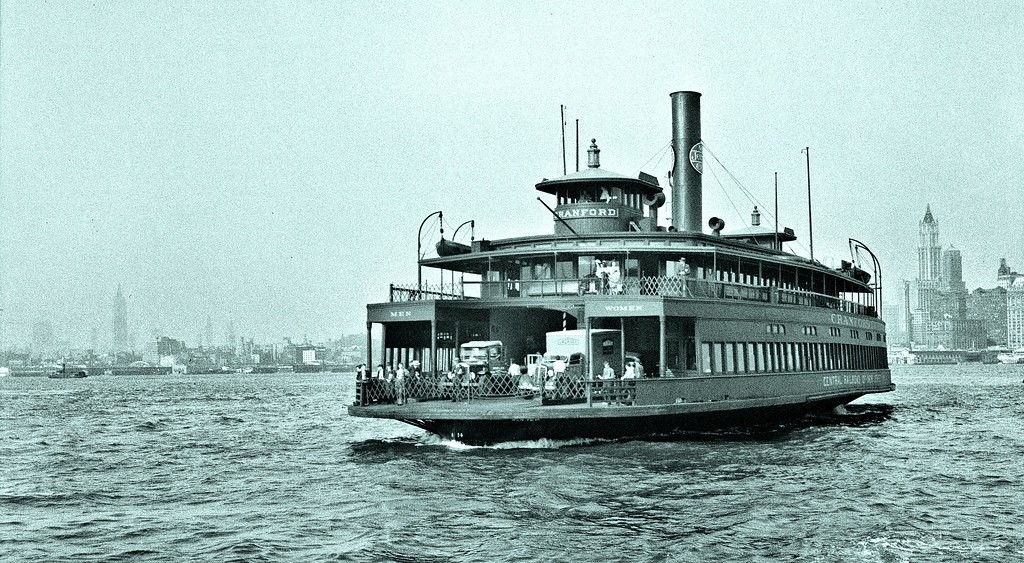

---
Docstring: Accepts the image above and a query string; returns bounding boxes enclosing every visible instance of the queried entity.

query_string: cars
[442,340,509,394]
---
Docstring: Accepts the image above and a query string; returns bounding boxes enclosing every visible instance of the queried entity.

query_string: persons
[449,358,463,402]
[594,259,622,295]
[356,360,421,406]
[553,356,566,400]
[598,361,615,405]
[620,358,644,406]
[677,258,690,296]
[508,360,521,393]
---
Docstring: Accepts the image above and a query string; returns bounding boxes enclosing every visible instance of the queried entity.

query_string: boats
[49,362,87,379]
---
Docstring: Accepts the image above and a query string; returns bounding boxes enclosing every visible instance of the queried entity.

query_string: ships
[348,89,898,447]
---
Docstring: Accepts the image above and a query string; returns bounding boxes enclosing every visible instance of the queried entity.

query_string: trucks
[517,327,623,399]
